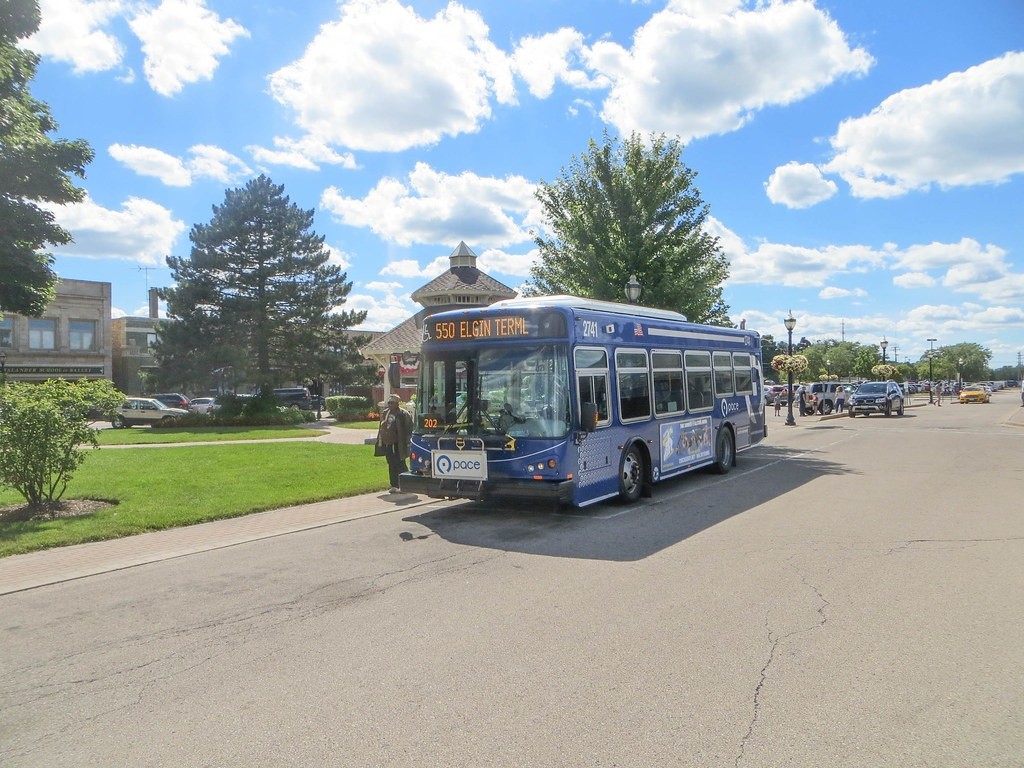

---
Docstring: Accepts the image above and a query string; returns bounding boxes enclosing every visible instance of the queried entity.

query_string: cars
[960,384,989,404]
[858,380,1019,396]
[310,394,325,412]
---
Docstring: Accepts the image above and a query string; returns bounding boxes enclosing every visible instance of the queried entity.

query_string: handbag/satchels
[374,433,386,455]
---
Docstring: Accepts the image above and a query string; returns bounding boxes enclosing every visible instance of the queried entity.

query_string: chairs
[661,390,712,411]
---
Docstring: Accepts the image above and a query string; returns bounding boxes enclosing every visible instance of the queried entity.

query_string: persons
[799,387,807,417]
[1020,380,1024,407]
[775,393,780,416]
[374,395,414,494]
[810,392,818,415]
[934,381,941,406]
[834,386,845,413]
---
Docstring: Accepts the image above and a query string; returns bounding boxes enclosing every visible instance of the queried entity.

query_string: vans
[253,387,314,411]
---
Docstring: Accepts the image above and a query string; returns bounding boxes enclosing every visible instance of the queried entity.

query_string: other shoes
[389,487,403,493]
[934,403,935,405]
[1020,405,1024,407]
[938,405,941,406]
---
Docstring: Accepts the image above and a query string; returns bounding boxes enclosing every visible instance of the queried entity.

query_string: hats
[813,392,817,395]
[387,394,401,402]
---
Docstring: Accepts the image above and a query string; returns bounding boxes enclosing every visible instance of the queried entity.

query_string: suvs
[764,379,808,406]
[149,392,256,420]
[111,397,190,429]
[792,382,861,414]
[846,379,905,418]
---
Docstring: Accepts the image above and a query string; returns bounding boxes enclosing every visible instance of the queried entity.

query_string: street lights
[784,308,797,425]
[827,360,831,382]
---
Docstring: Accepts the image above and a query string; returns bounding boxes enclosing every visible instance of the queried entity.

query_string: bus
[388,294,767,510]
